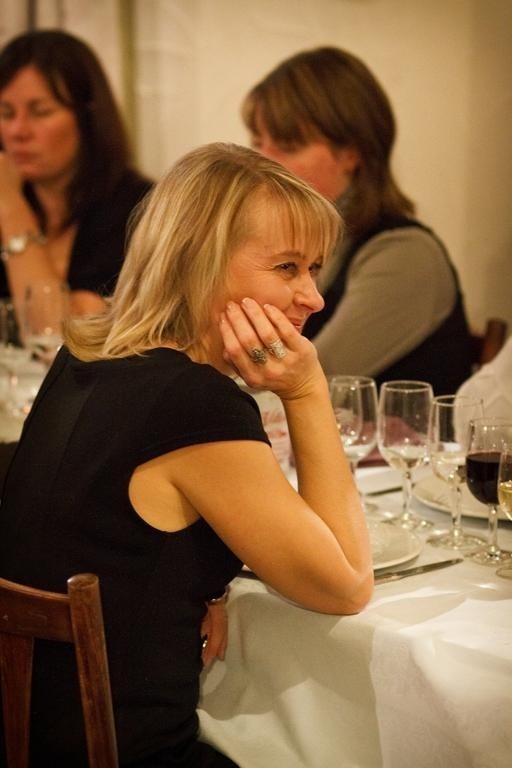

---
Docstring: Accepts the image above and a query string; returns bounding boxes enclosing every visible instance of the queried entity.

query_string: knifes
[374,558,463,585]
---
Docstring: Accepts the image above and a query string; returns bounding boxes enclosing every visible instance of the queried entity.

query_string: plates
[367,519,424,570]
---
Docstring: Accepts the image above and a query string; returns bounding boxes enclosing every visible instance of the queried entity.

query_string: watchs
[0,232,35,262]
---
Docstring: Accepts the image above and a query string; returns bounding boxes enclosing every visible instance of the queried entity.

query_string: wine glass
[330,376,378,515]
[0,281,70,419]
[376,381,512,580]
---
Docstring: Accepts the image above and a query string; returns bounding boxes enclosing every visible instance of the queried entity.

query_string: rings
[250,347,268,364]
[269,337,287,358]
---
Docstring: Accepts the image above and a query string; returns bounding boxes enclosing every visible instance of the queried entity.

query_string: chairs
[0,567,124,766]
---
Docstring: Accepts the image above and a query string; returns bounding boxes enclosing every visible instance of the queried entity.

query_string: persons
[0,29,158,365]
[239,46,470,447]
[0,141,376,768]
